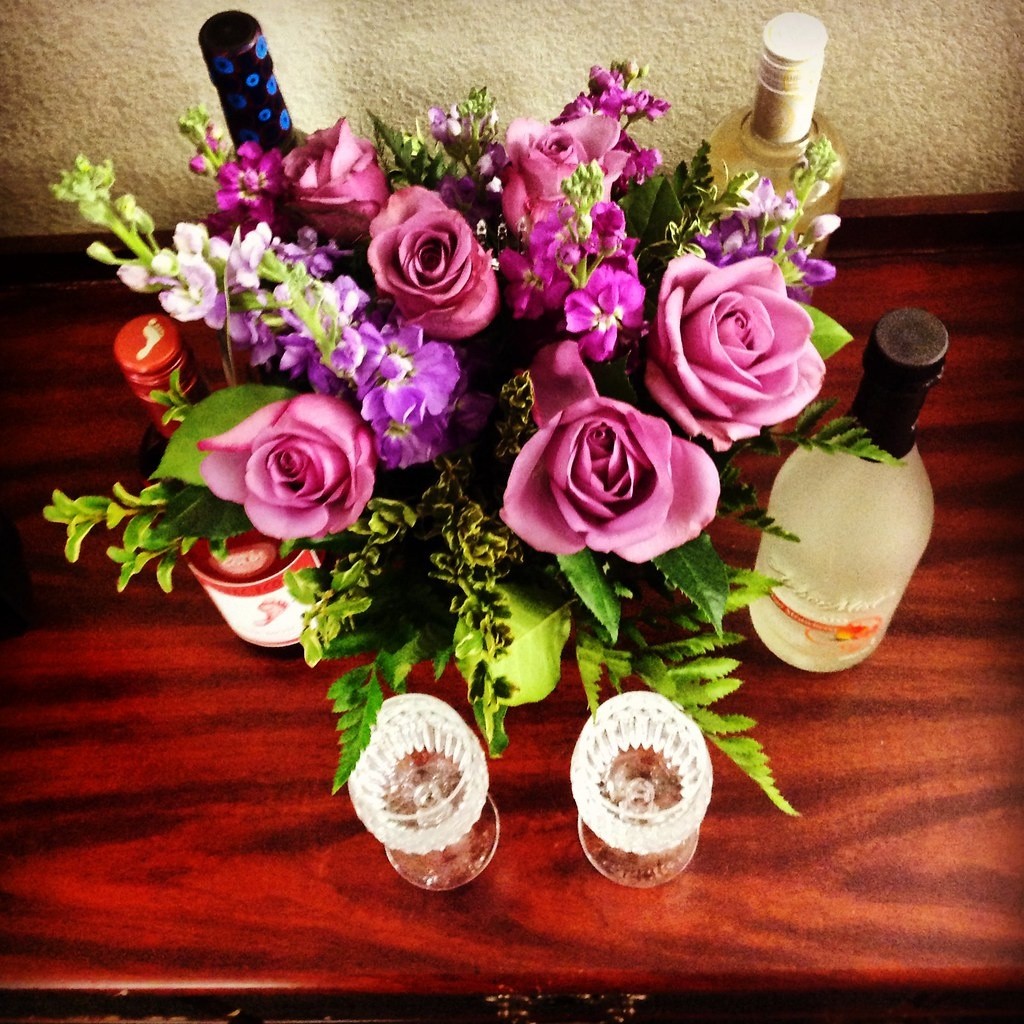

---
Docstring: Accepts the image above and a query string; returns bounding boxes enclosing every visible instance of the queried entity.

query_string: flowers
[42,57,902,819]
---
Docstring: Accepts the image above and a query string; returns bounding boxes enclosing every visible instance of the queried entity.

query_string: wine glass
[345,695,500,893]
[569,689,713,890]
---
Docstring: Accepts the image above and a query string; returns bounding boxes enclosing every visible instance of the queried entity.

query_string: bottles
[704,11,844,264]
[198,10,309,161]
[748,307,951,675]
[110,313,333,657]
